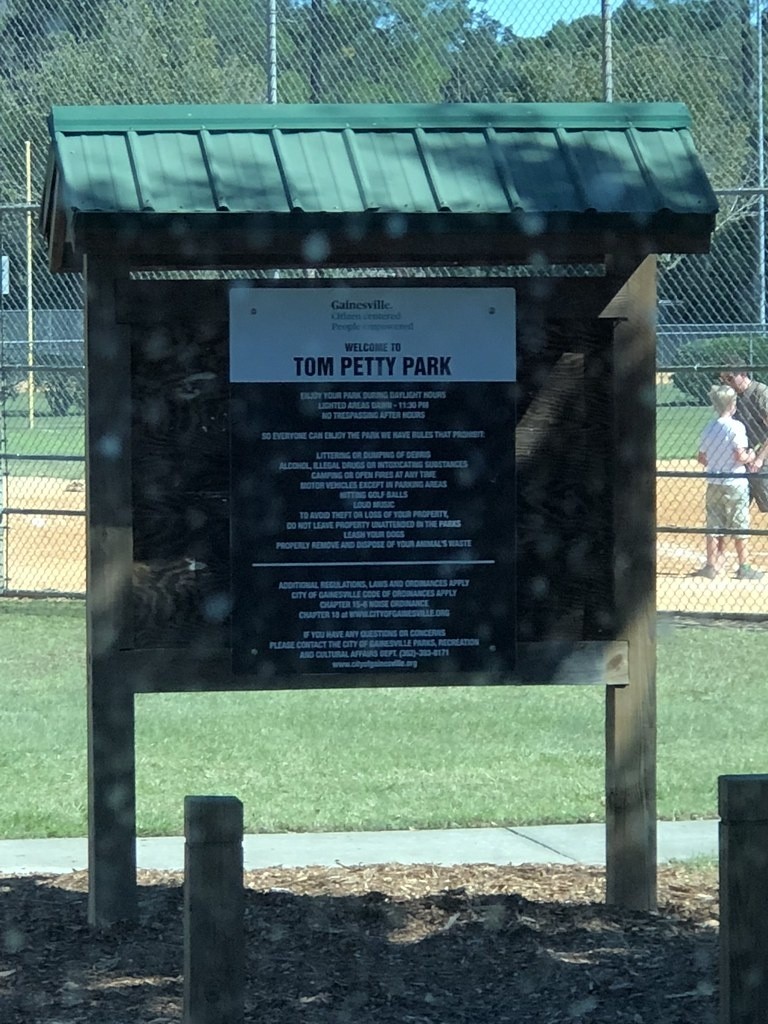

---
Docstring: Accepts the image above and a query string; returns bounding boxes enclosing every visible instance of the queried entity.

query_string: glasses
[717,376,738,385]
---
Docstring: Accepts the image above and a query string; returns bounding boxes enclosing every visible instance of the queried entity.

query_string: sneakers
[702,564,717,580]
[736,565,765,580]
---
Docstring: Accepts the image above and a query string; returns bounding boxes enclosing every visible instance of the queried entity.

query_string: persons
[716,357,768,580]
[696,384,765,580]
